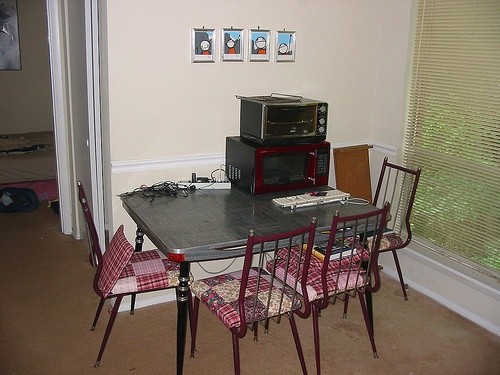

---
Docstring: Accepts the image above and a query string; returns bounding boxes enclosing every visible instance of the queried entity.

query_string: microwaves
[225,135,330,194]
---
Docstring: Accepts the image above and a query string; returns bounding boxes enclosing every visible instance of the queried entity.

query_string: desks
[120,185,392,375]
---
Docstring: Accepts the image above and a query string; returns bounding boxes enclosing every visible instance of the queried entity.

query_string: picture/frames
[273,30,297,62]
[221,27,245,62]
[248,28,272,62]
[191,27,216,62]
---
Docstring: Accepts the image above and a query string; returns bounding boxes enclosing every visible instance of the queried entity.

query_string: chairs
[190,217,317,375]
[327,157,421,302]
[266,201,391,375]
[77,181,197,369]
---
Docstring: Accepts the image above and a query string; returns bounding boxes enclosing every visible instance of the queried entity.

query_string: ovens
[240,94,328,145]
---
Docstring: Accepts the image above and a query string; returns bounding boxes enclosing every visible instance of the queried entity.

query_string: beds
[0,131,58,203]
